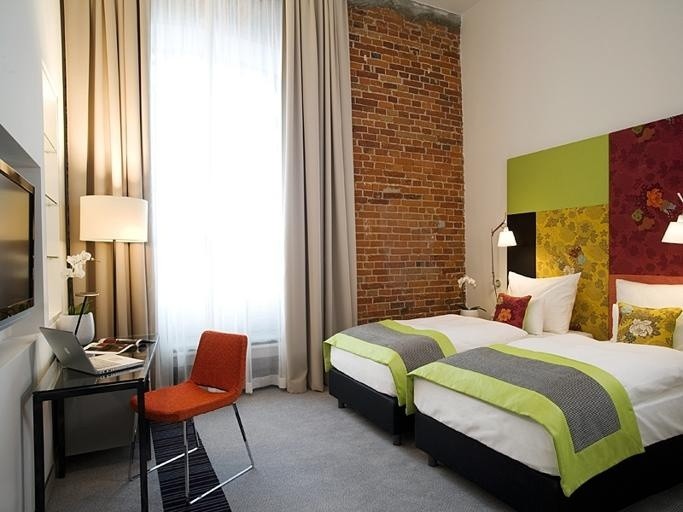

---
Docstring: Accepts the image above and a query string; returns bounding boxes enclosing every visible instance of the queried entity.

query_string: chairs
[128,331,254,506]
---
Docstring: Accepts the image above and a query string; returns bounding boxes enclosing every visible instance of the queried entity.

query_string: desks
[31,334,160,511]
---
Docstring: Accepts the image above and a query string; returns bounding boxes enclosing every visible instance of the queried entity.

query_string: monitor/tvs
[0,158,35,332]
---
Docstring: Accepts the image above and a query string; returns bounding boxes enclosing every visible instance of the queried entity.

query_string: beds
[406,273,683,512]
[321,313,593,446]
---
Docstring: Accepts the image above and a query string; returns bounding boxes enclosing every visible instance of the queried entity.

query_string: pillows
[614,279,682,349]
[616,303,682,349]
[610,302,618,343]
[521,295,548,336]
[507,270,581,336]
[492,293,532,329]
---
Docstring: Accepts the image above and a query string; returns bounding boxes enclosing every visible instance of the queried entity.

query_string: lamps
[78,195,147,340]
[660,192,682,245]
[488,214,517,295]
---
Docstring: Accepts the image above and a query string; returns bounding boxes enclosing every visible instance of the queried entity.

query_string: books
[82,339,156,354]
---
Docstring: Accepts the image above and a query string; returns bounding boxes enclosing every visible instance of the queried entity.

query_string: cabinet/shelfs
[43,132,58,260]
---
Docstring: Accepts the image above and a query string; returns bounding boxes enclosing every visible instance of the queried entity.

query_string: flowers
[63,250,91,315]
[454,275,488,313]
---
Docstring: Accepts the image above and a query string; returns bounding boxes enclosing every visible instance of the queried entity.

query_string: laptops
[40,326,145,375]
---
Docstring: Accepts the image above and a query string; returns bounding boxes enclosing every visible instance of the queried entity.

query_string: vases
[56,313,95,345]
[458,309,478,318]
